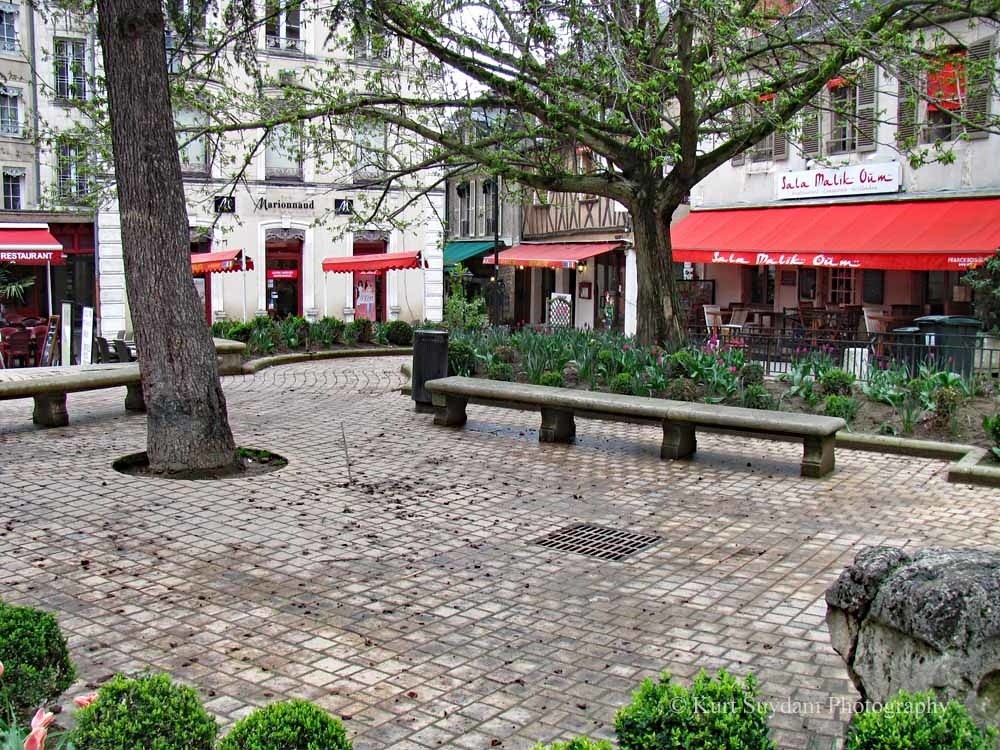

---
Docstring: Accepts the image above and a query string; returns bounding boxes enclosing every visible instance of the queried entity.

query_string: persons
[357,279,374,314]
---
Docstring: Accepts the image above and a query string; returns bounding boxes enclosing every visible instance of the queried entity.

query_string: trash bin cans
[914,316,984,378]
[892,326,921,381]
[412,329,449,408]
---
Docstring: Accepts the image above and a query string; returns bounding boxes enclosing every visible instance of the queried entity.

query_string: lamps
[577,261,584,275]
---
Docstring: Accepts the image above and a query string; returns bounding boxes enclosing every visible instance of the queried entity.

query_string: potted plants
[959,247,1000,369]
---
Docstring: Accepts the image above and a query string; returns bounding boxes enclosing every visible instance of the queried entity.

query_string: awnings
[0,224,66,265]
[670,195,1000,272]
[443,242,503,266]
[191,248,254,326]
[482,242,623,269]
[322,249,425,325]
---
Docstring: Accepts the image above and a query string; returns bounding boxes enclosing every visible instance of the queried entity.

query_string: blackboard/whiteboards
[39,315,60,366]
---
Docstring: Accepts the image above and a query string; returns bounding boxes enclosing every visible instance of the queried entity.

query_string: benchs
[424,375,845,479]
[0,362,147,426]
[212,337,245,376]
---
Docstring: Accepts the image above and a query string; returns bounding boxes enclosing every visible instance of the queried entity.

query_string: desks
[98,340,137,357]
[868,314,910,354]
[706,308,785,332]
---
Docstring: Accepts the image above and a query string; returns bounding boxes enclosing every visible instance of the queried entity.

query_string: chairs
[94,329,134,362]
[0,311,48,368]
[703,300,897,359]
